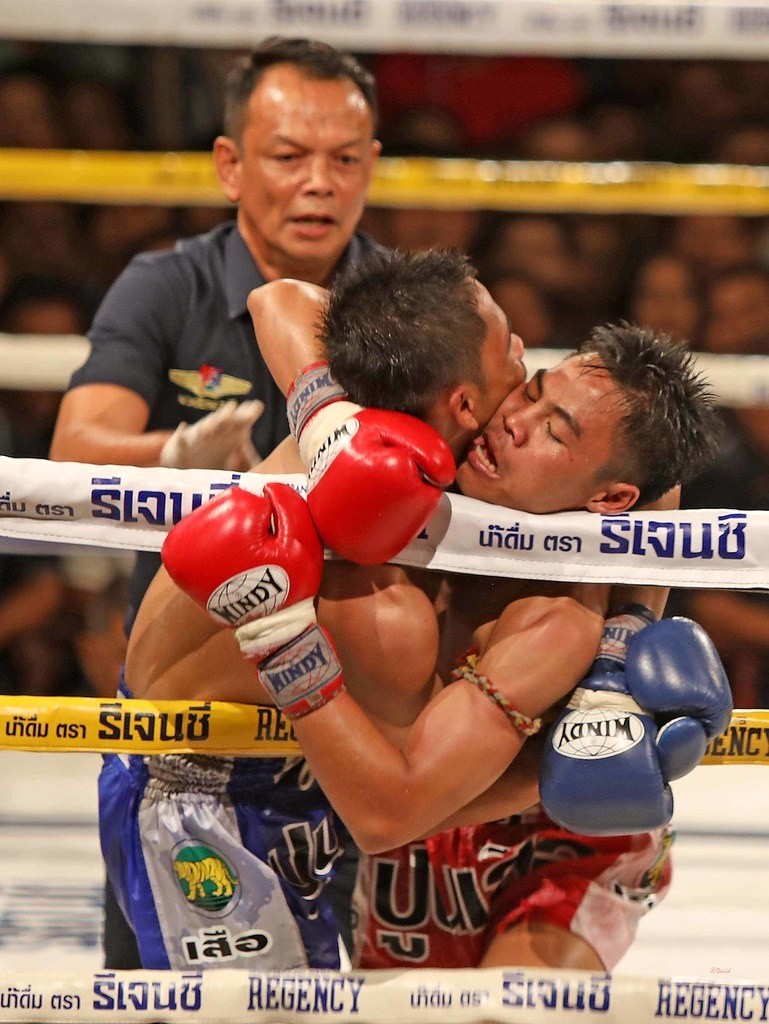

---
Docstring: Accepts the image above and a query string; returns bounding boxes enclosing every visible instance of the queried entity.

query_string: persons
[97,247,737,969]
[0,34,769,709]
[49,34,392,968]
[160,279,723,968]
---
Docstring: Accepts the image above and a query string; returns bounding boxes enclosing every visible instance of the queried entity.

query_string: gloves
[162,480,327,655]
[537,612,675,837]
[298,397,458,565]
[622,615,731,785]
